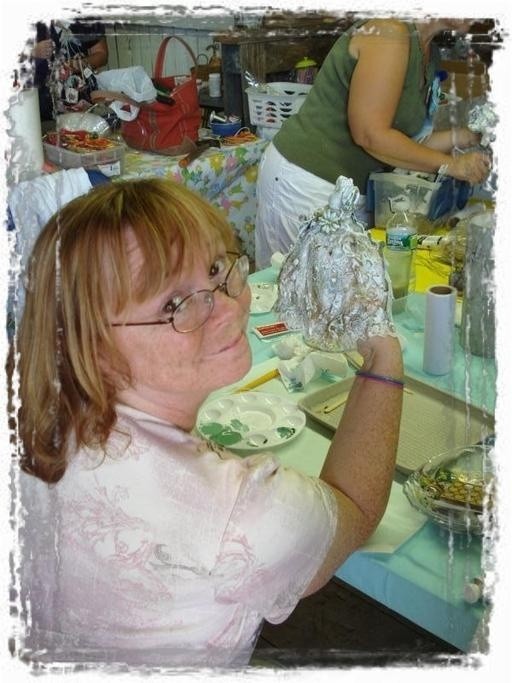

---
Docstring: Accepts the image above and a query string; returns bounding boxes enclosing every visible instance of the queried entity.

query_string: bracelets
[354,371,406,387]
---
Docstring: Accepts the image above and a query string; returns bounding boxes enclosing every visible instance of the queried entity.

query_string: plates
[403,443,494,536]
[195,391,307,453]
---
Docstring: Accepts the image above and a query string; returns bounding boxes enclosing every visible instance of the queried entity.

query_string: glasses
[105,252,250,334]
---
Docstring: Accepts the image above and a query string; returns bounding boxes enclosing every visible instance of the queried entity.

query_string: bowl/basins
[211,122,242,136]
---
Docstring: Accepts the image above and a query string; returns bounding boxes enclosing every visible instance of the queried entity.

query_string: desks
[110,118,266,263]
[193,259,484,652]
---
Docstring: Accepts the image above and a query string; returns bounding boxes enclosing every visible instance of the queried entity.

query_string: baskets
[245,82,313,128]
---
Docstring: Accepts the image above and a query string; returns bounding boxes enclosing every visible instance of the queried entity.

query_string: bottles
[381,199,415,315]
[208,74,222,98]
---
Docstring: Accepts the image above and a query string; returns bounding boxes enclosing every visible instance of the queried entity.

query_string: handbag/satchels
[90,36,202,156]
[94,65,157,121]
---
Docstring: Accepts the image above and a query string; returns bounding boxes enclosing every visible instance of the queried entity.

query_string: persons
[253,18,491,269]
[32,20,108,119]
[12,179,405,665]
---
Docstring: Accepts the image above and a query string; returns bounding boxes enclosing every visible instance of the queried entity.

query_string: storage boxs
[368,170,441,229]
